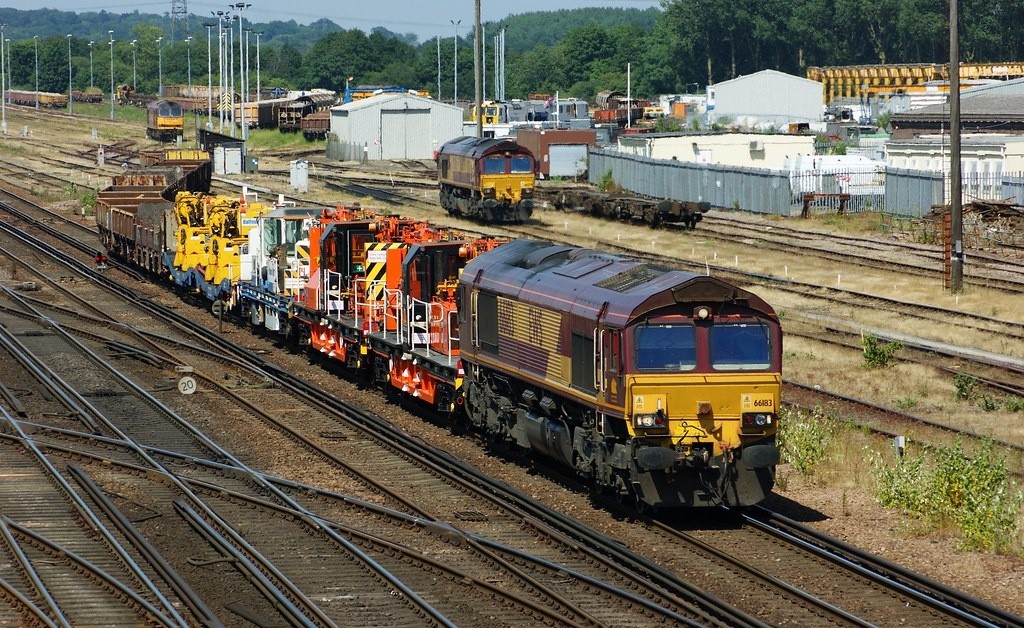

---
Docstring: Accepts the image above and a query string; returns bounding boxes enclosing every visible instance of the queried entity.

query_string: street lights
[480,22,489,102]
[34,36,39,109]
[253,31,265,101]
[185,37,193,99]
[130,40,137,91]
[0,23,8,130]
[5,39,11,104]
[88,41,94,88]
[109,31,116,119]
[67,34,72,113]
[229,2,252,140]
[202,23,217,131]
[451,20,461,108]
[211,11,230,134]
[156,37,163,97]
[243,27,254,102]
[492,25,509,101]
[220,15,238,138]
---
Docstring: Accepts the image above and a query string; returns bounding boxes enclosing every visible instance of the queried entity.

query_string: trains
[74,86,104,103]
[235,89,340,140]
[93,148,784,511]
[5,89,67,108]
[436,135,536,224]
[472,96,666,139]
[116,85,217,114]
[146,100,184,142]
